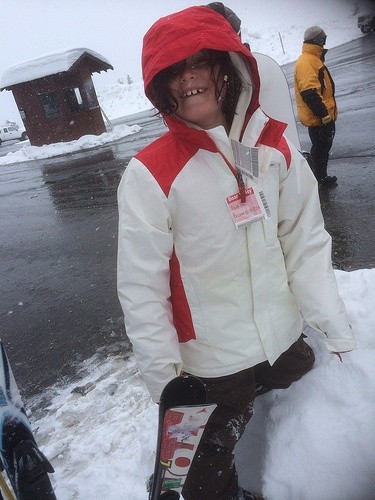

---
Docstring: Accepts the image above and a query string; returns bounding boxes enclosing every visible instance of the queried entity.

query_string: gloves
[320,114,335,139]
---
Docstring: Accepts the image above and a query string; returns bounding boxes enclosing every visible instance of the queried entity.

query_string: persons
[295,25,338,189]
[116,2,357,500]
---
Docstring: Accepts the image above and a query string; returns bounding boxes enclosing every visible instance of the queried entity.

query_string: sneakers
[319,174,338,184]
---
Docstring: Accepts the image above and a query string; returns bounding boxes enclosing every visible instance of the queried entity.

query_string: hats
[303,25,327,44]
